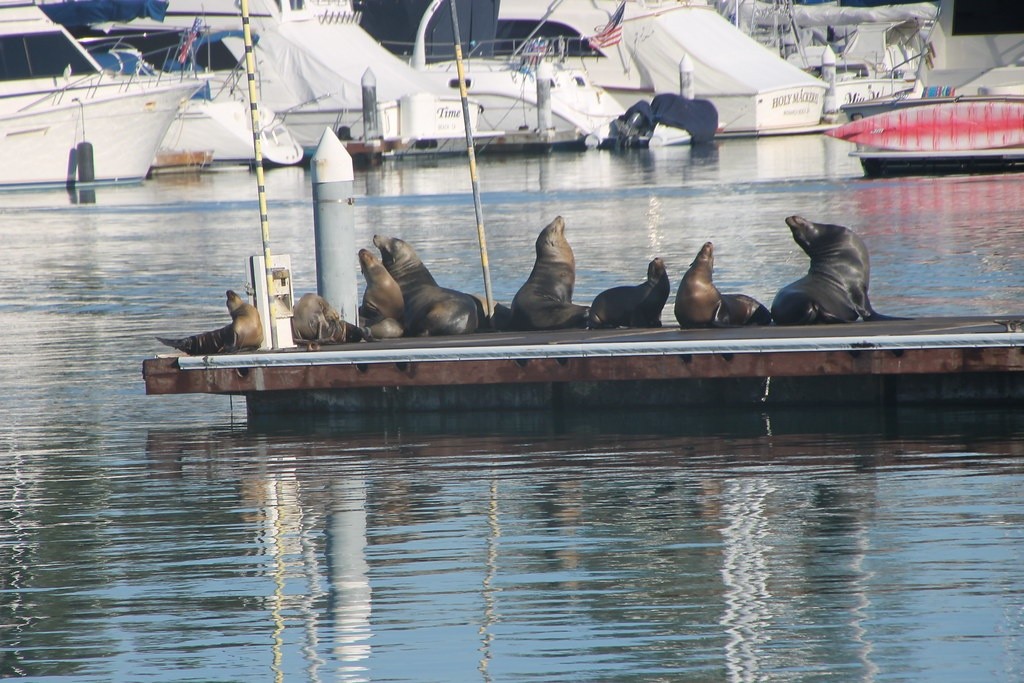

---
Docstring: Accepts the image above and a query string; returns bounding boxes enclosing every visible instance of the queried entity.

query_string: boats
[821,57,1024,178]
[0,2,944,193]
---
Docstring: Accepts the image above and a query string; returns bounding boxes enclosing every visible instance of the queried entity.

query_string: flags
[178,18,202,64]
[589,1,626,48]
